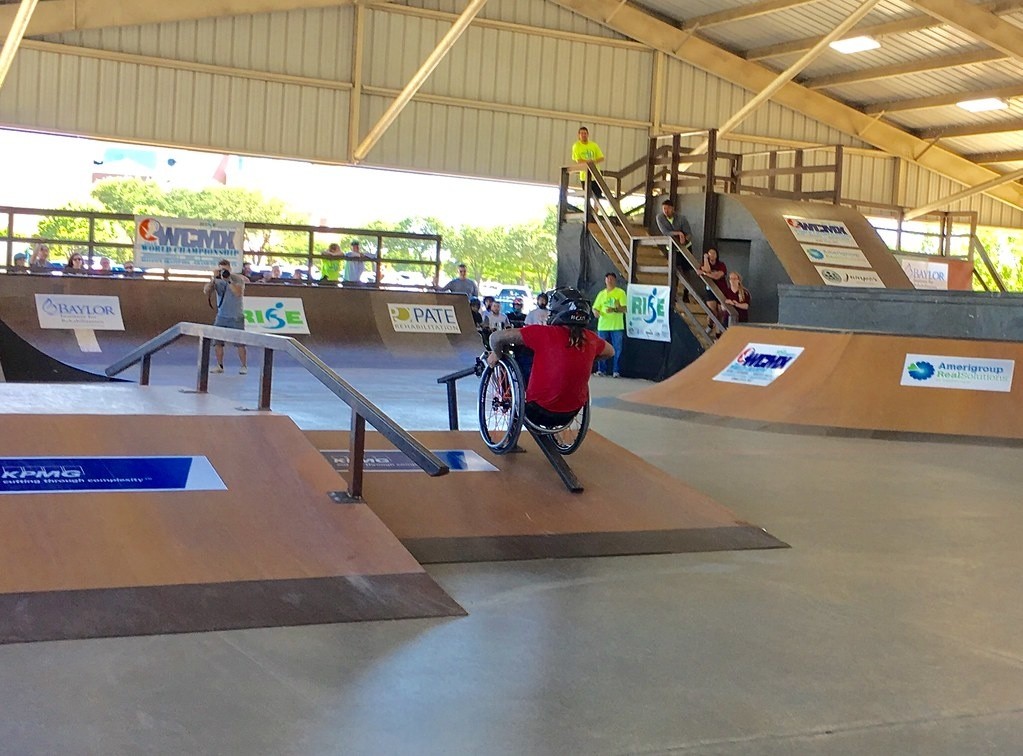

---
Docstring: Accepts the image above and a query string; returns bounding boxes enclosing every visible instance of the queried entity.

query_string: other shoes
[210,366,225,374]
[714,334,720,342]
[593,371,607,377]
[239,365,249,374]
[613,371,620,378]
[705,325,713,335]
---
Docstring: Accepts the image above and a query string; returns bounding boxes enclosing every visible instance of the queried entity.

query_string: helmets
[547,285,592,325]
[469,296,480,309]
[512,297,524,313]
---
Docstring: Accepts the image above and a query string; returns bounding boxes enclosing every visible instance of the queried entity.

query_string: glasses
[73,257,82,261]
[730,277,738,281]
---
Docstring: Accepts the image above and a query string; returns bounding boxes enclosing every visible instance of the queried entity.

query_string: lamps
[956,95,1010,113]
[829,33,883,55]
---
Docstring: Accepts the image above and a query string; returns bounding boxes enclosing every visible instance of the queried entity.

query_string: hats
[606,272,616,278]
[14,252,26,260]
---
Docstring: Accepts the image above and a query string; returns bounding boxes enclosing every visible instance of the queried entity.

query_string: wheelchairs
[473,337,592,454]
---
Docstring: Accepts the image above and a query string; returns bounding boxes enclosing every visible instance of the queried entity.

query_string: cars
[480,282,533,313]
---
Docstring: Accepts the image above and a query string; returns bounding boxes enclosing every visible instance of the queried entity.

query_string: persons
[695,247,751,343]
[288,268,305,285]
[62,252,88,277]
[430,263,479,304]
[571,126,606,216]
[486,285,616,438]
[235,262,286,284]
[340,240,372,288]
[97,257,118,278]
[469,292,552,351]
[6,252,30,275]
[655,199,694,303]
[202,258,249,375]
[316,243,345,287]
[591,271,627,379]
[122,259,144,278]
[28,237,56,276]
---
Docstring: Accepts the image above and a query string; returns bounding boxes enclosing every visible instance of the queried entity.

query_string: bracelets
[703,271,707,275]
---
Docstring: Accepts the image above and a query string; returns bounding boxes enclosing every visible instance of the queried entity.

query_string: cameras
[216,269,230,279]
